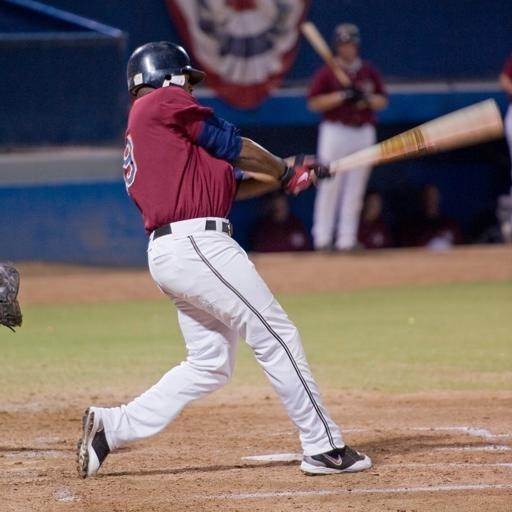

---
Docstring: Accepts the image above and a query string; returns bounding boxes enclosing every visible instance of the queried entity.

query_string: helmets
[127,42,206,91]
[333,23,360,45]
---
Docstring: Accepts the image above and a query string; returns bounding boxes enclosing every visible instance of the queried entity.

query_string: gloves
[279,150,331,196]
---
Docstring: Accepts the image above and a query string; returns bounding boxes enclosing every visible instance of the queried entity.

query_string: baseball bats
[316,98,503,173]
[300,20,353,91]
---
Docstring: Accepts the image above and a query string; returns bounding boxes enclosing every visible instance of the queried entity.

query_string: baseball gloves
[1,263,22,332]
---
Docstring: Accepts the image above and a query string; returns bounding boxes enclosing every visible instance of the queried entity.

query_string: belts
[152,221,234,239]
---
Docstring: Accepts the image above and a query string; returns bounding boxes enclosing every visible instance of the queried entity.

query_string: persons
[69,39,375,480]
[253,185,511,249]
[302,19,397,253]
[499,41,512,152]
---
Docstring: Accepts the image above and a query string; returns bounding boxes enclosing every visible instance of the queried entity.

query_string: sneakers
[300,445,373,476]
[76,408,109,478]
